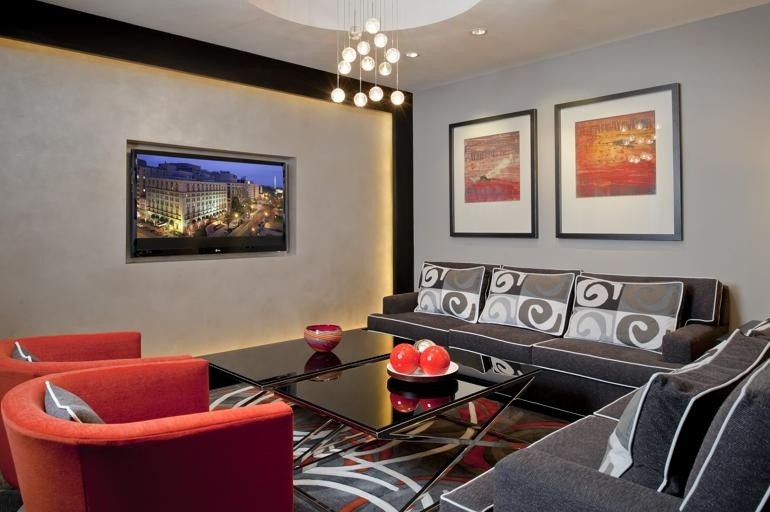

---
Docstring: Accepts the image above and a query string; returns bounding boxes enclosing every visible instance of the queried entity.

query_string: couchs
[0,358,295,512]
[365,259,732,412]
[439,319,770,511]
[0,331,192,493]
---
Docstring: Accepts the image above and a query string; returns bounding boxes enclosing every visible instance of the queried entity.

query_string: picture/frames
[449,108,539,238]
[554,82,683,240]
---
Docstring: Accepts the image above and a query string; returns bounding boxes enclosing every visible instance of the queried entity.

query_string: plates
[387,361,459,377]
[387,377,458,399]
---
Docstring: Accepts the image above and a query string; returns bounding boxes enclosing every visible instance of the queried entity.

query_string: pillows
[479,267,575,337]
[746,318,770,342]
[598,329,770,492]
[563,275,685,355]
[11,341,40,364]
[42,380,105,423]
[413,263,485,325]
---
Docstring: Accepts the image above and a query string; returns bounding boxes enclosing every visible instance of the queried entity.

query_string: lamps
[331,18,405,106]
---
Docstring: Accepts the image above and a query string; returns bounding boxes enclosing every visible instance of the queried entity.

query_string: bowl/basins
[304,324,342,353]
[304,352,342,382]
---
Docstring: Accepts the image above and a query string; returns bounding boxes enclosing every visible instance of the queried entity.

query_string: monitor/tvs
[130,149,290,257]
